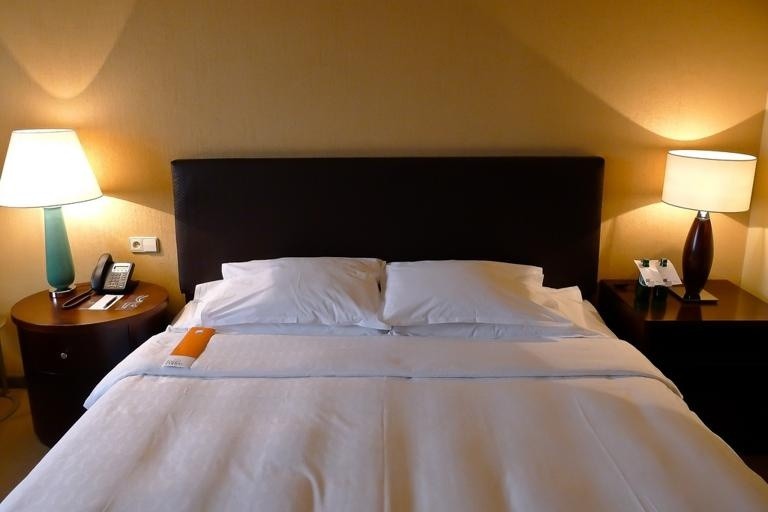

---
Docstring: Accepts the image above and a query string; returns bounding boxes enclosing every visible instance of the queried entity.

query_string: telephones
[90,253,135,292]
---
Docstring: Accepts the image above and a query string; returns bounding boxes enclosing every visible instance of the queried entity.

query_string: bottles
[635,259,650,299]
[653,258,669,301]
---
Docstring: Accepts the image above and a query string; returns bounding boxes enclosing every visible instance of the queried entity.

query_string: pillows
[379,259,574,328]
[204,258,393,331]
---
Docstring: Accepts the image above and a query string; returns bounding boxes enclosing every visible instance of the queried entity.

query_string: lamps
[660,147,757,305]
[0,125,103,298]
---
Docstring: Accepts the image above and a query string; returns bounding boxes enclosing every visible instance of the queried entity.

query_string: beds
[0,159,768,511]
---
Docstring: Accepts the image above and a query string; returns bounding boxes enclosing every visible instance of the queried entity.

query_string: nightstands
[594,275,767,457]
[10,280,176,457]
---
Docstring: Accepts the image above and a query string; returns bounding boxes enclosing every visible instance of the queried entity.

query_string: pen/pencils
[104,296,117,308]
